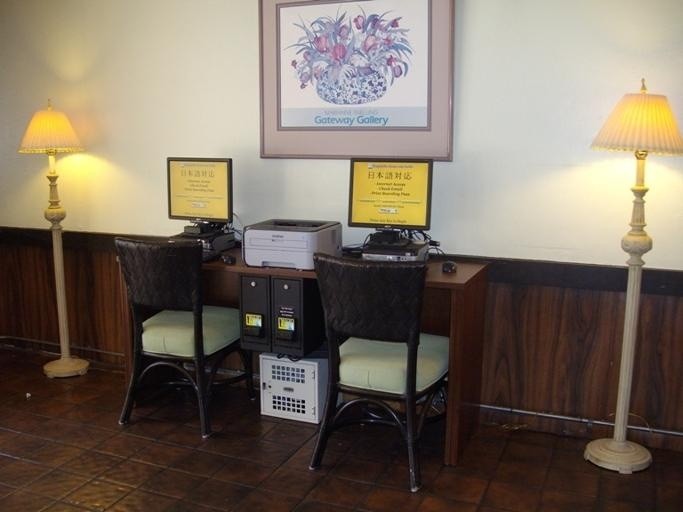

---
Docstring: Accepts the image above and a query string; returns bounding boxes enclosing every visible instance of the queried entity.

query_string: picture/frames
[259,0,454,162]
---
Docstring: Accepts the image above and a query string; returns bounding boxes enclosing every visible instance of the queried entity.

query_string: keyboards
[202,253,218,263]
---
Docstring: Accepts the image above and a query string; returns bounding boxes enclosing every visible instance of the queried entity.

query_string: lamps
[583,80,682,476]
[20,100,89,377]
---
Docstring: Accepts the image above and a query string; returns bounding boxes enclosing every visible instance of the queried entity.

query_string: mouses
[220,254,236,265]
[443,261,457,272]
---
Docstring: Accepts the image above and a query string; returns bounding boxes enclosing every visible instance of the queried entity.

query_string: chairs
[115,239,253,438]
[309,254,449,493]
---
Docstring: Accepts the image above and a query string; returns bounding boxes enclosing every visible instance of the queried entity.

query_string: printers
[241,219,342,270]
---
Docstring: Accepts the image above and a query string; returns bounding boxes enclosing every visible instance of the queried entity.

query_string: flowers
[284,6,412,87]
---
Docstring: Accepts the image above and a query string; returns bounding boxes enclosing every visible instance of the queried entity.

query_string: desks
[117,247,489,465]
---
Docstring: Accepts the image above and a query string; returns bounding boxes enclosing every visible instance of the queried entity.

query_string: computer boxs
[362,240,430,261]
[168,232,236,253]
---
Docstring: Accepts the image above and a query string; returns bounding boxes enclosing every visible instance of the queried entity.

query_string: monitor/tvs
[349,157,433,247]
[167,157,232,237]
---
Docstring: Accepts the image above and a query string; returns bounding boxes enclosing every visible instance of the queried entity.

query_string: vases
[316,65,387,105]
